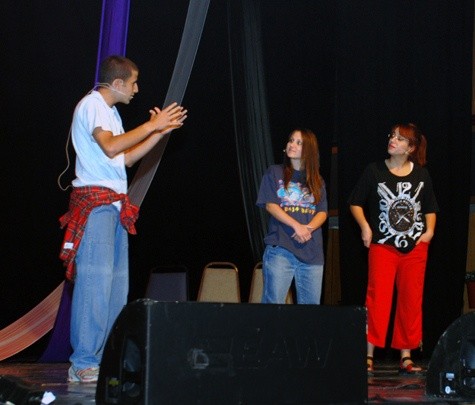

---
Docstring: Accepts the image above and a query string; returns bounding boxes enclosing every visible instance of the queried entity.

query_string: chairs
[249,261,293,305]
[196,262,242,302]
[146,264,190,300]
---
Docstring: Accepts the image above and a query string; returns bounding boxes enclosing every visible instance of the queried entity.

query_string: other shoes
[69,363,100,382]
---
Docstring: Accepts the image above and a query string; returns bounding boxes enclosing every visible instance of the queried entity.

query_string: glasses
[388,132,410,142]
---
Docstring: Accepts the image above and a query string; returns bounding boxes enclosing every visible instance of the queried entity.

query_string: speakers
[95,298,368,405]
[423,309,475,405]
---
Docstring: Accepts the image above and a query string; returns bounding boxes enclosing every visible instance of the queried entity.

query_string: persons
[58,56,187,385]
[256,128,328,306]
[348,124,437,372]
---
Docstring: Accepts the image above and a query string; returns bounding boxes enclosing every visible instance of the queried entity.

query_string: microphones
[97,82,134,99]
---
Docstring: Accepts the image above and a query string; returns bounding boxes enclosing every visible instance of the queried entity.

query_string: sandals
[366,355,375,375]
[399,357,422,374]
